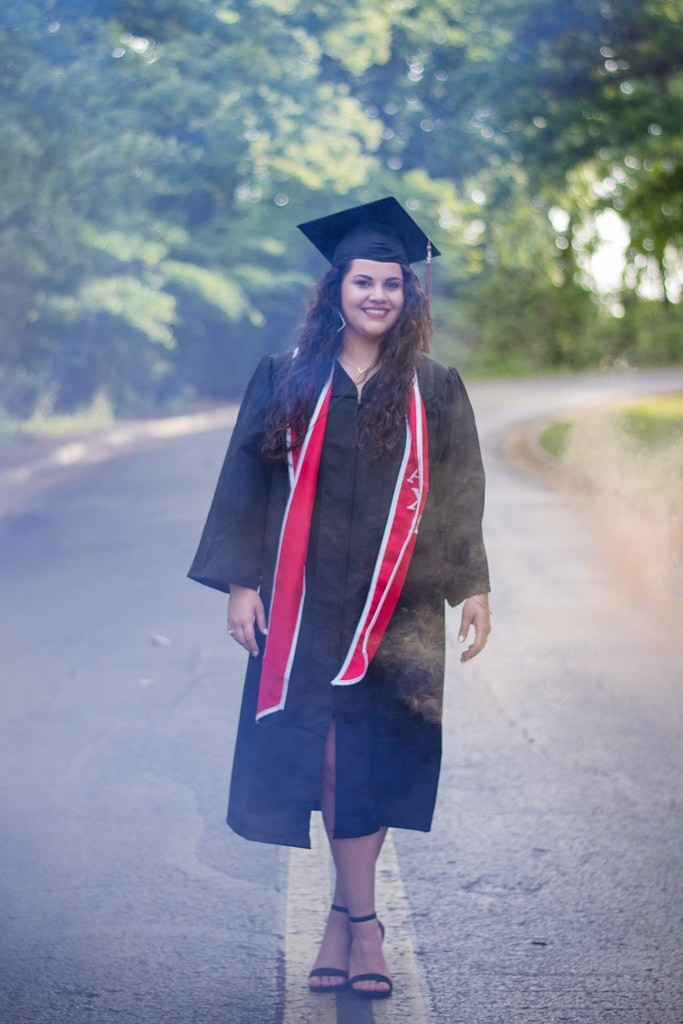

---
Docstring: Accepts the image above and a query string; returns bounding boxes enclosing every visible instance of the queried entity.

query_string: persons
[186,196,493,998]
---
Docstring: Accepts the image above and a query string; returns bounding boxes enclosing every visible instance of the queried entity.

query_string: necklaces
[339,355,366,373]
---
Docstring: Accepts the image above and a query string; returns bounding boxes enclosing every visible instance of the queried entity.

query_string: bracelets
[471,596,493,615]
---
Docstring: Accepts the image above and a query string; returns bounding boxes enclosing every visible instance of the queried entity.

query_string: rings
[227,630,234,635]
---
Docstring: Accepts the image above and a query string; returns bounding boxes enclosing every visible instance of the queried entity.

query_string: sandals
[309,904,349,992]
[349,912,392,998]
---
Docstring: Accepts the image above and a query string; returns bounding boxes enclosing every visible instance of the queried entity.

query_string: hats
[297,196,442,353]
